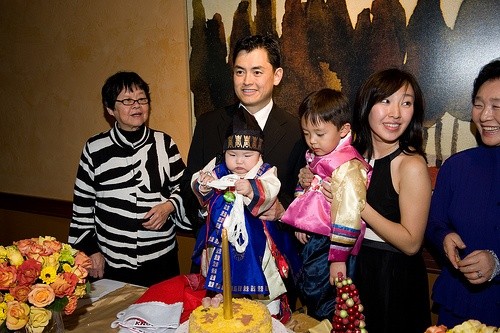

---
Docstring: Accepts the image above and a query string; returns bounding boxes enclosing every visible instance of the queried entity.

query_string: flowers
[0,236,93,333]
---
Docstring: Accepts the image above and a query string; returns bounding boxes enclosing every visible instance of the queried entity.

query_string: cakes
[189,297,272,333]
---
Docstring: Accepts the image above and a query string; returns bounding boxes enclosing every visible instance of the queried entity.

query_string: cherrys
[330,272,365,333]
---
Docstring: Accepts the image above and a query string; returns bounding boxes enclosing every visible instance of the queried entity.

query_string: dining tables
[64,278,192,332]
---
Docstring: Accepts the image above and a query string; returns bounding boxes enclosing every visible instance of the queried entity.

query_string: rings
[476,270,482,278]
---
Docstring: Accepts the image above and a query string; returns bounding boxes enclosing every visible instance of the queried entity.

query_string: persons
[423,59,500,332]
[190,119,297,321]
[298,68,432,333]
[68,71,195,287]
[281,87,373,322]
[179,33,309,312]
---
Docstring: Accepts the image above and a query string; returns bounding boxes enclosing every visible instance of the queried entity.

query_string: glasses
[116,97,149,106]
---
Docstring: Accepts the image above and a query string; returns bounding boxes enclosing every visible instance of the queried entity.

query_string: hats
[223,122,263,154]
